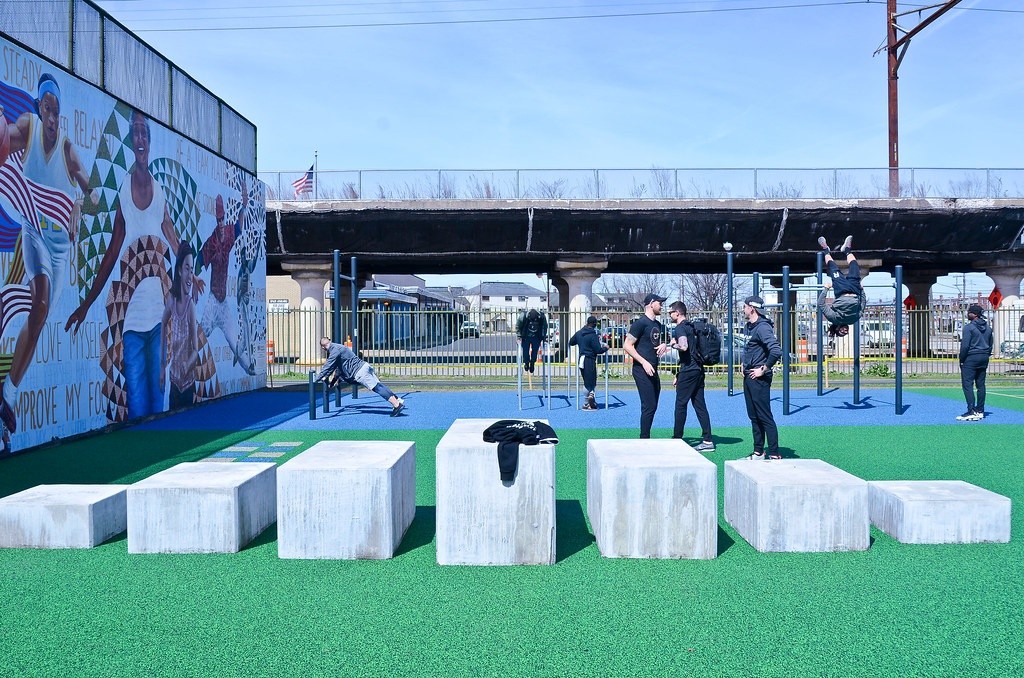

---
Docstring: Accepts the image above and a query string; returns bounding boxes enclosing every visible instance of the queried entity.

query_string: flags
[292,164,314,200]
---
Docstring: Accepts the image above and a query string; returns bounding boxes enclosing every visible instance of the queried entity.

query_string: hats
[529,310,539,322]
[745,296,766,315]
[643,294,668,307]
[588,316,599,324]
[969,305,987,319]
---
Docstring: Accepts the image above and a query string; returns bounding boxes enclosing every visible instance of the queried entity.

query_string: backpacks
[682,318,721,366]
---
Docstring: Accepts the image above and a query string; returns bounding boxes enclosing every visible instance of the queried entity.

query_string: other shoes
[530,362,534,373]
[524,362,529,371]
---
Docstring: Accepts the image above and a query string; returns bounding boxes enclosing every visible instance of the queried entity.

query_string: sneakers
[956,411,977,422]
[742,452,766,460]
[977,412,984,418]
[769,456,782,460]
[582,404,591,410]
[840,236,853,251]
[390,403,405,417]
[393,399,404,409]
[694,441,715,452]
[818,237,830,251]
[588,394,597,409]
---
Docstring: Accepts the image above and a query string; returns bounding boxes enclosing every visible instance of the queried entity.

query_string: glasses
[669,311,676,314]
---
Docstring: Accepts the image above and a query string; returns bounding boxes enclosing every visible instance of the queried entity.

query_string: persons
[515,309,548,373]
[622,293,667,439]
[670,300,716,452]
[817,236,866,338]
[955,306,994,421]
[569,316,609,411]
[740,296,783,461]
[315,337,404,417]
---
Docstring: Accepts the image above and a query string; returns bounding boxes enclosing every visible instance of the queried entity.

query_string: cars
[722,331,752,366]
[460,320,479,338]
[603,327,627,346]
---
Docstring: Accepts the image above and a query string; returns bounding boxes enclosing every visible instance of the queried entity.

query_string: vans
[798,320,837,359]
[860,320,895,348]
[544,319,560,337]
[952,318,992,342]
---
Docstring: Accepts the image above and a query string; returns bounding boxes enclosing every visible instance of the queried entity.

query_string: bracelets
[760,365,763,371]
[671,343,675,349]
